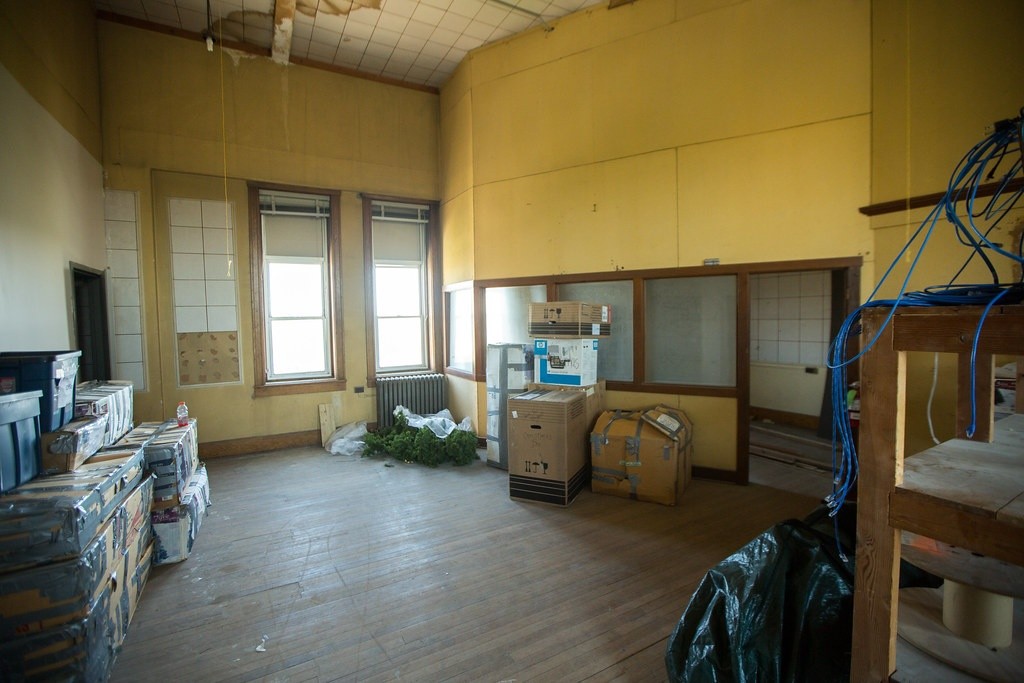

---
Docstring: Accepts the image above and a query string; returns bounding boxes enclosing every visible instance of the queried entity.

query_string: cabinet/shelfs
[849,292,1024,683]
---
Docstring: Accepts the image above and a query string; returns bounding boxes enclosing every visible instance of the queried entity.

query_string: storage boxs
[504,301,692,508]
[0,349,212,683]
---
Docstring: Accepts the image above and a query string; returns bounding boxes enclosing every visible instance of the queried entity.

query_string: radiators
[375,374,447,429]
